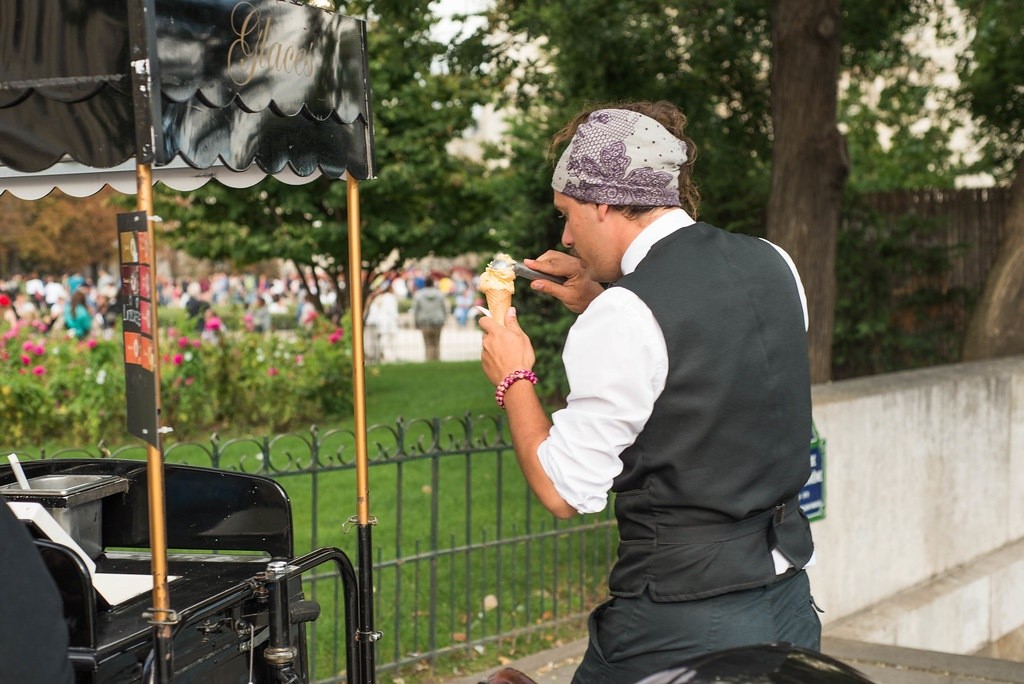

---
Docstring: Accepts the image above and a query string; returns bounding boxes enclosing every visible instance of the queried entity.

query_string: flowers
[1,291,346,390]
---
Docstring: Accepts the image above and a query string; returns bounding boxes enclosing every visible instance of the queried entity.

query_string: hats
[551,108,687,208]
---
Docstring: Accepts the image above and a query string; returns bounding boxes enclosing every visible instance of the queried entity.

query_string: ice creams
[478,267,515,328]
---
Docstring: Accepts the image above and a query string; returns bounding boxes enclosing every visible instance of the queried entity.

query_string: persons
[0,253,485,359]
[478,100,823,684]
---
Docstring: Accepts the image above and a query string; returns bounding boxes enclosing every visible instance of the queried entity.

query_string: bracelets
[495,369,539,409]
